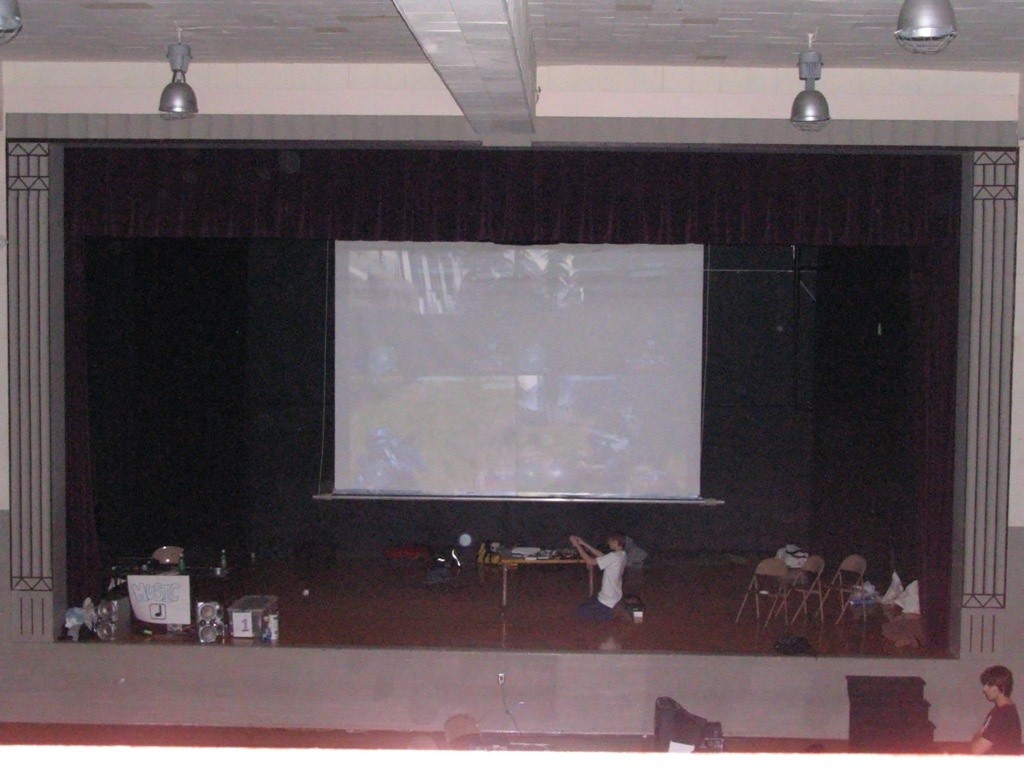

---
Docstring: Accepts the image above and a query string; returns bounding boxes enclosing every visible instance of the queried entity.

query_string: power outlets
[498,673,504,686]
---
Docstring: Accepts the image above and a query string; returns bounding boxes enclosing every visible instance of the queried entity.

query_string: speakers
[196,601,223,644]
[98,595,130,641]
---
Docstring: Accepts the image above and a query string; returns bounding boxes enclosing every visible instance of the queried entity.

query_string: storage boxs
[227,595,278,638]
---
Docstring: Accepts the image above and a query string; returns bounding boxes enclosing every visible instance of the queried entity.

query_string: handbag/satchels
[653,694,722,751]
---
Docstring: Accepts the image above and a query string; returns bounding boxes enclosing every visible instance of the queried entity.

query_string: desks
[500,555,594,606]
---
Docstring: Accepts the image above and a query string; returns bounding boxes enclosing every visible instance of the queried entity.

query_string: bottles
[178,553,186,575]
[220,549,228,569]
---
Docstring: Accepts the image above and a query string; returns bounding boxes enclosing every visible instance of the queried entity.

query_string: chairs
[736,559,789,628]
[775,555,824,622]
[407,735,439,750]
[443,713,486,750]
[815,555,867,625]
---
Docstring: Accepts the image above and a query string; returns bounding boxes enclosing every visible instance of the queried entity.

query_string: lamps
[791,35,830,132]
[157,28,198,120]
[894,0,959,55]
[0,0,23,44]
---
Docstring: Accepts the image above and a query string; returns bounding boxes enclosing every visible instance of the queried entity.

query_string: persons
[970,666,1022,753]
[621,534,647,612]
[568,529,629,621]
